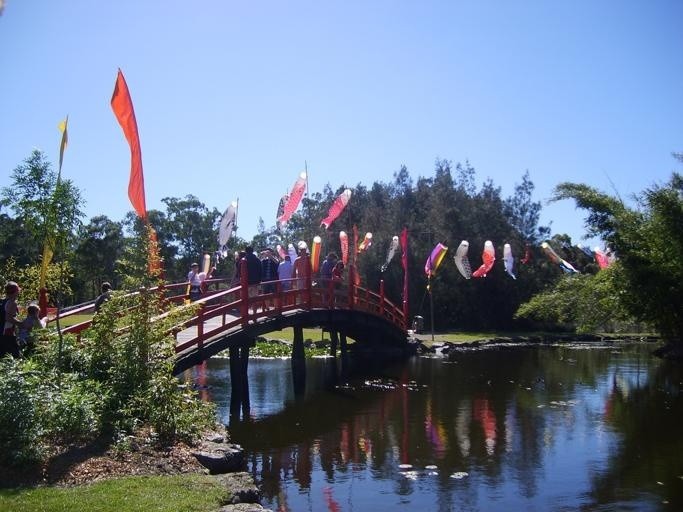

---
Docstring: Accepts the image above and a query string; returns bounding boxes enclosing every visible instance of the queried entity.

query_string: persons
[187,262,205,302]
[229,244,345,310]
[0,281,23,361]
[92,281,112,316]
[18,305,48,354]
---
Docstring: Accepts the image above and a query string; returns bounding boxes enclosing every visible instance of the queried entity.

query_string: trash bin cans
[412,315,424,333]
